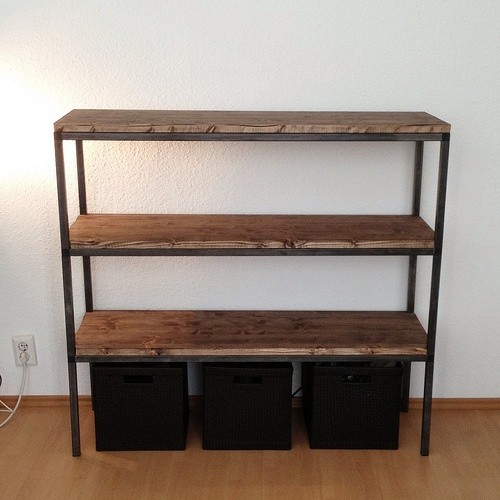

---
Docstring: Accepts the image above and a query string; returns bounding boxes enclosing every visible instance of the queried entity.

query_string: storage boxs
[302,361,401,451]
[89,363,189,452]
[202,362,294,451]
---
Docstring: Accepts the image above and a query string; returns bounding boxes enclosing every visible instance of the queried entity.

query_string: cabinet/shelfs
[53,108,452,456]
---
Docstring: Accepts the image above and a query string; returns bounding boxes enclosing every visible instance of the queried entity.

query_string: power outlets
[12,335,38,367]
